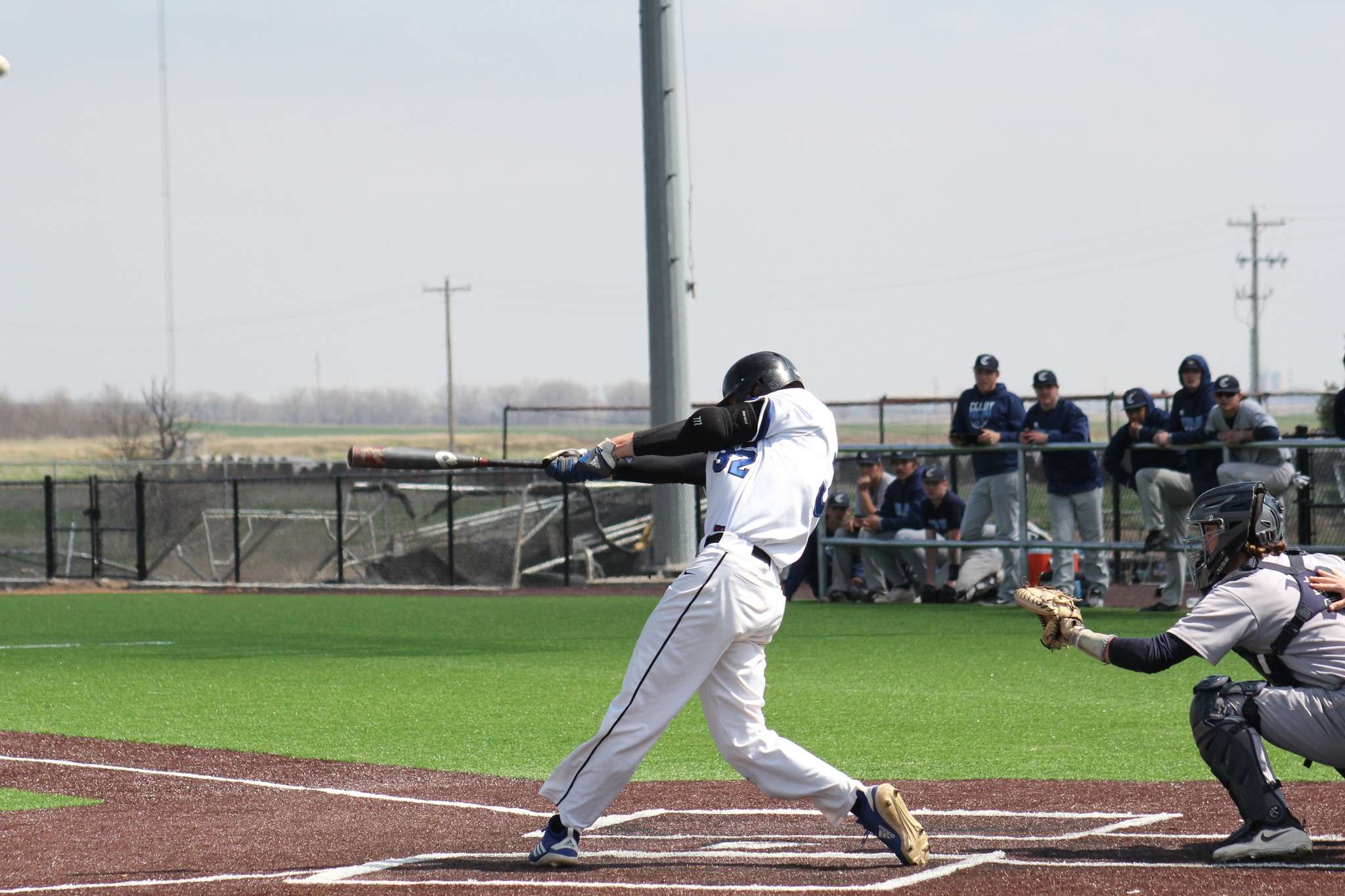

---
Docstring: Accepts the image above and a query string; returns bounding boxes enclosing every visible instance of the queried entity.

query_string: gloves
[543,448,589,483]
[577,437,635,479]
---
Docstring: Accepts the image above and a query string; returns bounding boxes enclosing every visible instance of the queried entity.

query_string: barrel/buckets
[1026,548,1078,588]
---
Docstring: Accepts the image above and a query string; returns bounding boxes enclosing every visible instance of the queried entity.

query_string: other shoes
[1140,599,1182,612]
[1078,591,1104,608]
[825,580,1019,607]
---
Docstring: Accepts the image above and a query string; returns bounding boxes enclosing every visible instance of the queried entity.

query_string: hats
[857,451,881,463]
[829,492,850,507]
[1120,389,1147,412]
[973,354,998,371]
[922,462,944,483]
[1182,359,1201,371]
[890,450,917,459]
[1213,375,1239,392]
[1032,370,1057,386]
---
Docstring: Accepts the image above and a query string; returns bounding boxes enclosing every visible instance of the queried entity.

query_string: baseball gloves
[1013,579,1085,654]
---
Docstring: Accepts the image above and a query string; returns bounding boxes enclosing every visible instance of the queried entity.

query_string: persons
[526,351,932,870]
[784,353,1345,859]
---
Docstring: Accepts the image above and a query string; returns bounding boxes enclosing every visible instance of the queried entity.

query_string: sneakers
[856,783,930,865]
[1027,521,1051,542]
[1212,816,1313,860]
[528,814,580,865]
[1141,530,1169,555]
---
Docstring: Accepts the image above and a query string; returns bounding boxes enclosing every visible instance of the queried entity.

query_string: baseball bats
[347,444,545,470]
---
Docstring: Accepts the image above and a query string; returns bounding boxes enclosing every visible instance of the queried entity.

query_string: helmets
[1183,481,1285,590]
[715,351,805,405]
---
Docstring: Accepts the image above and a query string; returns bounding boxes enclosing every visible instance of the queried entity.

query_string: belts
[695,534,771,566]
